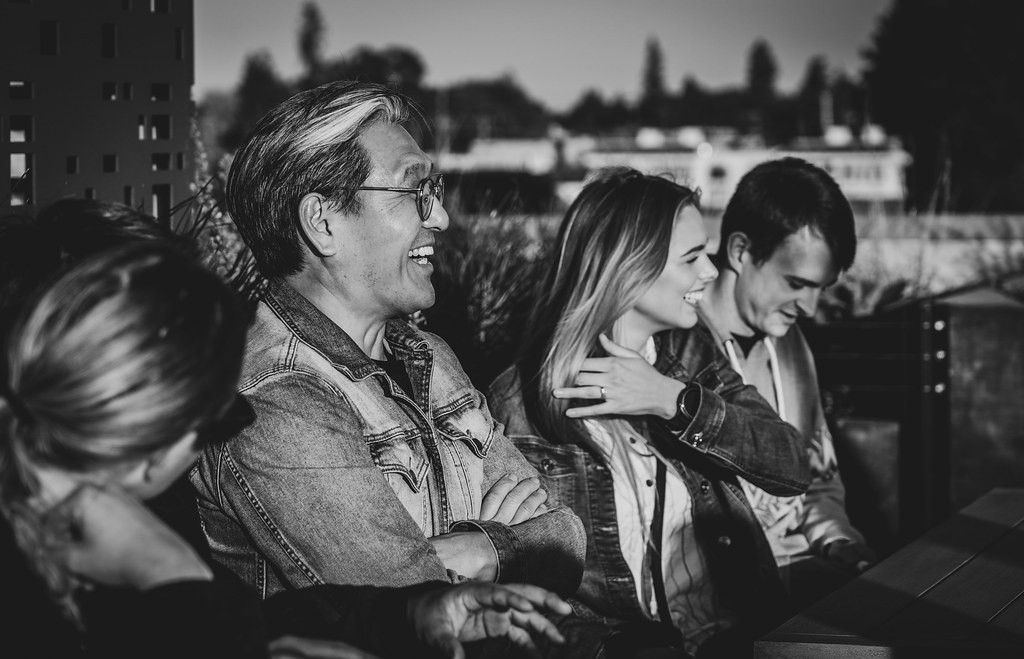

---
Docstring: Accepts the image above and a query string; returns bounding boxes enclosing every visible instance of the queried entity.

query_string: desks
[757,485,1024,658]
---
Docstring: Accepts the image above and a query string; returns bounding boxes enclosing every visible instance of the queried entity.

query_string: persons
[488,167,811,659]
[696,157,880,605]
[185,79,690,659]
[0,197,574,659]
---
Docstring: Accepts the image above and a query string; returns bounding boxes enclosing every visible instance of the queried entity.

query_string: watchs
[664,382,699,432]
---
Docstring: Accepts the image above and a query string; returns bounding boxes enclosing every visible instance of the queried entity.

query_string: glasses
[314,172,445,222]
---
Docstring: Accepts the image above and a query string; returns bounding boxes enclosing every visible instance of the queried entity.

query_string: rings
[601,386,605,399]
